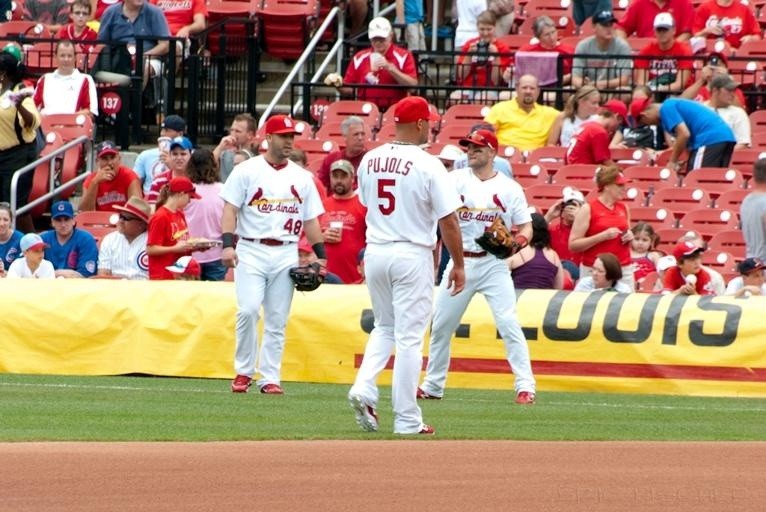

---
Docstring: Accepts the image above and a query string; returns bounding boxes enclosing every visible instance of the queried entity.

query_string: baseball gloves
[288,262,325,291]
[474,212,519,259]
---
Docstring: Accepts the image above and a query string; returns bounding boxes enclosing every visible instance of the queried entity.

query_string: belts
[244,236,289,245]
[460,252,486,257]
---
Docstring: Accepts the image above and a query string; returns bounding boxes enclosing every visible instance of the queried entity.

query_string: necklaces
[391,136,414,147]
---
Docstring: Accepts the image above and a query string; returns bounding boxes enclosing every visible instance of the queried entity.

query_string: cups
[330,221,344,243]
[158,137,171,157]
[370,51,382,72]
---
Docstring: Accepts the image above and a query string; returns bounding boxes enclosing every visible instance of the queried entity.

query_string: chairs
[0,0,765,288]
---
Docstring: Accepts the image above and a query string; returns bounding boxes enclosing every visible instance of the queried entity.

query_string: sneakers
[393,422,435,435]
[262,385,283,393]
[515,392,534,403]
[232,375,253,393]
[416,387,441,399]
[348,394,380,431]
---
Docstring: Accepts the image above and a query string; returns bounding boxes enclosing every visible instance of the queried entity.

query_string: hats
[266,115,302,135]
[672,240,705,261]
[394,96,442,124]
[562,192,583,205]
[367,17,391,40]
[596,170,634,192]
[19,116,201,276]
[592,8,743,127]
[330,159,354,174]
[459,129,498,154]
[740,257,765,275]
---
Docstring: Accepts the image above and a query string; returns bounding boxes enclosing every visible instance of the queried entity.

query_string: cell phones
[711,56,718,66]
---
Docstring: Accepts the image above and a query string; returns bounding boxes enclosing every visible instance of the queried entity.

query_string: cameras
[477,40,488,65]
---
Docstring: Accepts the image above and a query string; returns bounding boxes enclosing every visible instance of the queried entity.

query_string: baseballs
[686,274,697,286]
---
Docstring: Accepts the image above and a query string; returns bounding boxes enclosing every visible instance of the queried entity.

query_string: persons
[411,128,539,406]
[345,95,466,432]
[1,1,766,296]
[220,113,329,393]
